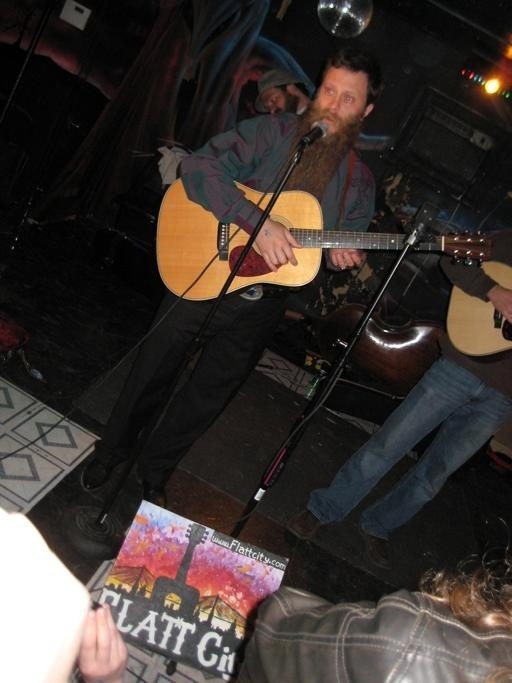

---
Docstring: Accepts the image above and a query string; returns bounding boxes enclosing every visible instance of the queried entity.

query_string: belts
[238,283,282,300]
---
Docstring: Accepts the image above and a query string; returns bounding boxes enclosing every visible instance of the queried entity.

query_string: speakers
[379,86,512,211]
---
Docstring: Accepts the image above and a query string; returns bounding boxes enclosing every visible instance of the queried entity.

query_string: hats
[253,71,305,114]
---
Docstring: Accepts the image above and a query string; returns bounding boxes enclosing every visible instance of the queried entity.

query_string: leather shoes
[358,519,392,570]
[80,457,112,492]
[286,507,322,540]
[136,464,166,508]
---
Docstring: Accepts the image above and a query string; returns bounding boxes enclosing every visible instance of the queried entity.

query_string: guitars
[155,175,493,302]
[446,260,511,358]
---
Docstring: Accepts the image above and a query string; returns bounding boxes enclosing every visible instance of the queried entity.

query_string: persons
[234,517,511,683]
[80,45,388,517]
[257,68,311,117]
[285,227,512,570]
[1,507,129,683]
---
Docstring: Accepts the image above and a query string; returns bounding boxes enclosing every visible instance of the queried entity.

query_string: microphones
[294,120,328,152]
[411,201,442,229]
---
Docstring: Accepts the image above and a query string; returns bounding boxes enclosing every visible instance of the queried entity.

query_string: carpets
[72,345,441,594]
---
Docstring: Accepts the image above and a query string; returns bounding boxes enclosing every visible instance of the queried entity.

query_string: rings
[341,266,346,270]
